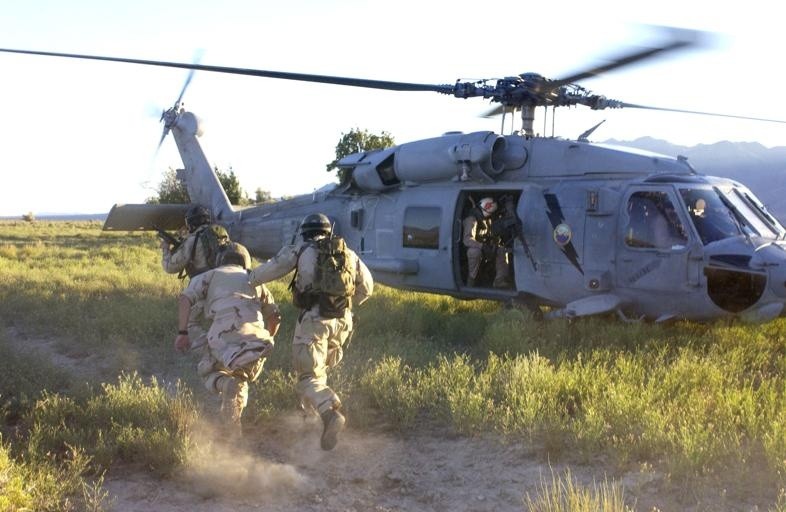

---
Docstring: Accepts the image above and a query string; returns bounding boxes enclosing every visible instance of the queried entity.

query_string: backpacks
[192,225,230,268]
[304,237,355,297]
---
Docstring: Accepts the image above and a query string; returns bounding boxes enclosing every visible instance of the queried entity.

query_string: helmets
[300,213,333,234]
[477,197,499,218]
[185,204,211,221]
[220,242,251,271]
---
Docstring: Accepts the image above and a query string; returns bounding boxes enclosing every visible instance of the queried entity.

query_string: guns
[468,196,496,248]
[504,196,538,270]
[152,223,183,252]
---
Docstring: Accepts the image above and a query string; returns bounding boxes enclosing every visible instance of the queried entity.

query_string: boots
[320,408,345,451]
[216,375,243,425]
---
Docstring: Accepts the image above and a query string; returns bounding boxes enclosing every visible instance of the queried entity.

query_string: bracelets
[178,330,188,335]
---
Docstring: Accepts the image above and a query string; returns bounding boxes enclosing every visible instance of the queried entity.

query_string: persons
[247,212,374,450]
[160,204,232,350]
[462,196,513,289]
[174,242,282,444]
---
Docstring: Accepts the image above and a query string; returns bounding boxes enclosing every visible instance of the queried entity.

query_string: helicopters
[0,28,785,328]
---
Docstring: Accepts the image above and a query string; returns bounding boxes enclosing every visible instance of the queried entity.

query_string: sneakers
[494,276,514,288]
[467,274,479,287]
